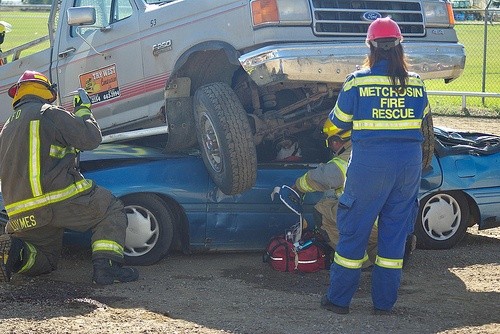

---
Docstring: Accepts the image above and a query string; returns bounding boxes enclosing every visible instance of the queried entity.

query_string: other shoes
[402,234,417,269]
[320,295,350,314]
[93,258,139,284]
[374,305,394,315]
[0,234,23,282]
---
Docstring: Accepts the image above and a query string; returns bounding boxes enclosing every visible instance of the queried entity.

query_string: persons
[0,72,138,285]
[290,117,417,274]
[274,138,305,163]
[0,20,12,66]
[323,18,431,315]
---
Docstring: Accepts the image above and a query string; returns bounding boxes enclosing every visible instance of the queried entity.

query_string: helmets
[366,18,404,40]
[0,22,7,33]
[323,117,352,147]
[8,70,57,103]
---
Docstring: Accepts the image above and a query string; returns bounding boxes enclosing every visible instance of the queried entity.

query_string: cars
[0,1,467,198]
[0,120,500,270]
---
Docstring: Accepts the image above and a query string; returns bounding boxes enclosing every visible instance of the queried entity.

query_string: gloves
[73,88,92,108]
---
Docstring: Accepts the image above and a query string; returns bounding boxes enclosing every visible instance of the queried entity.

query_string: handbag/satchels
[263,230,331,272]
